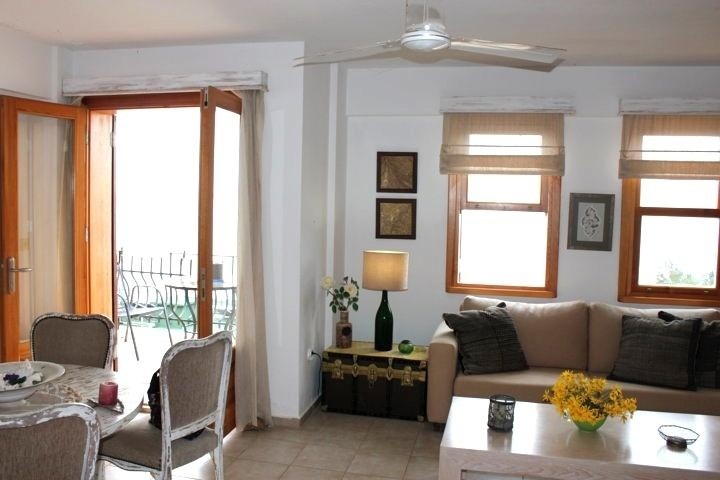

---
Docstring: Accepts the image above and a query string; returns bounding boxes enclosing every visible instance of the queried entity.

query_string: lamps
[361,250,410,352]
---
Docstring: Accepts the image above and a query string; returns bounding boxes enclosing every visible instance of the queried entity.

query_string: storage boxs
[320,341,429,422]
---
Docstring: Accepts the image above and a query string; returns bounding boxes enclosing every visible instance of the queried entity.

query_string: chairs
[118,285,174,360]
[28,311,116,370]
[0,402,102,480]
[97,331,233,480]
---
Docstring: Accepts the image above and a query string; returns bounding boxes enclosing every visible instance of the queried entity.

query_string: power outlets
[308,348,316,361]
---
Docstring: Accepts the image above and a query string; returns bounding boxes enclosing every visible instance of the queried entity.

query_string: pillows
[461,295,589,372]
[605,314,703,393]
[442,302,530,374]
[658,309,719,390]
[591,301,718,373]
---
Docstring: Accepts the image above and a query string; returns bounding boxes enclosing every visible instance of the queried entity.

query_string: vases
[573,418,607,432]
[335,312,353,348]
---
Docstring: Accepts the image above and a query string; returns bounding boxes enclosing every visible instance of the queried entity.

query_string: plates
[1,360,65,403]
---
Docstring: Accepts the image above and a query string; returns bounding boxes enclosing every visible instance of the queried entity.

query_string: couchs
[426,296,719,431]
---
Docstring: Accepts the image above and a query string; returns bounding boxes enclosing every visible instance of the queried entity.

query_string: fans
[293,2,567,68]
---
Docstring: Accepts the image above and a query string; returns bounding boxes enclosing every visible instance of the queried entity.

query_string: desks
[165,281,237,339]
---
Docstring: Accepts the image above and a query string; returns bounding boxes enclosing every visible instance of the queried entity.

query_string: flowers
[542,370,638,423]
[320,275,361,313]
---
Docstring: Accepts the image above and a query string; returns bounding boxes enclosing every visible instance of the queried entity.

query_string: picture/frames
[374,197,417,240]
[376,150,418,193]
[567,192,615,252]
